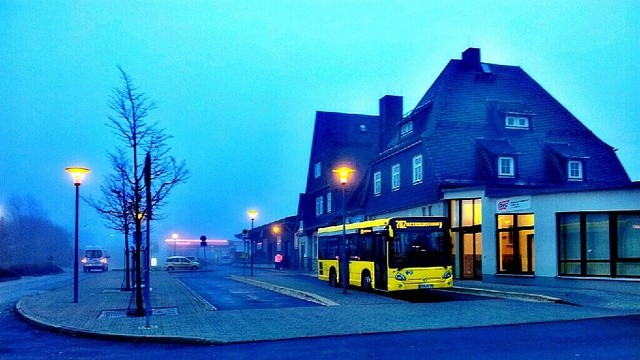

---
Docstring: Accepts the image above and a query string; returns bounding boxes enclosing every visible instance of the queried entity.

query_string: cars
[185,256,198,262]
[221,255,231,265]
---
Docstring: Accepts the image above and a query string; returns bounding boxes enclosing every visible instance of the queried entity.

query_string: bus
[317,216,454,294]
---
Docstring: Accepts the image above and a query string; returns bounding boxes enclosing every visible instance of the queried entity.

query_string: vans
[82,245,110,271]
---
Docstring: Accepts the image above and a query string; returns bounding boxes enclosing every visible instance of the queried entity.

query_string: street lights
[246,209,258,277]
[332,165,356,294]
[64,164,91,302]
[172,233,178,256]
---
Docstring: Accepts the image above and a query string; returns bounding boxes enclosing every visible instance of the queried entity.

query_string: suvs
[164,255,201,273]
